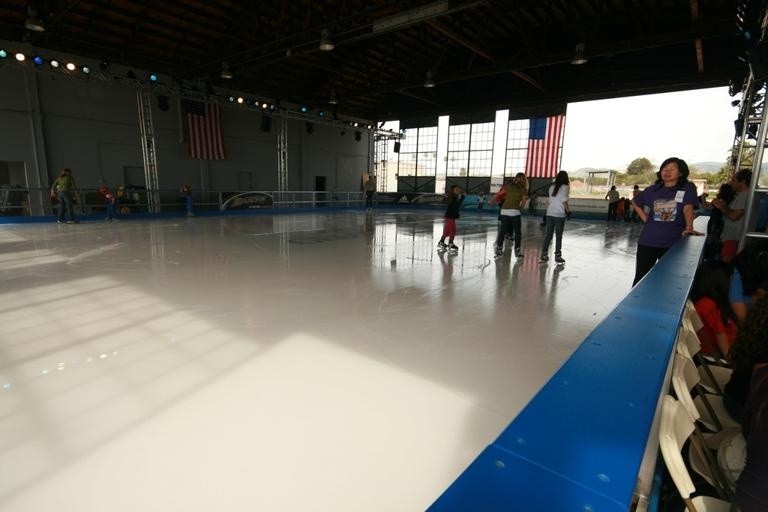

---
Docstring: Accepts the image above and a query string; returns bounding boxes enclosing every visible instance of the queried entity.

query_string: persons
[363,176,376,209]
[535,263,565,311]
[496,262,523,299]
[690,169,768,512]
[606,185,644,223]
[438,186,465,249]
[632,157,704,287]
[52,168,78,224]
[438,250,458,300]
[100,186,120,224]
[477,171,571,263]
[180,184,194,217]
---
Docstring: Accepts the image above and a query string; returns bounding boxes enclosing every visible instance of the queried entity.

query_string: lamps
[424,70,435,88]
[572,42,588,64]
[221,61,233,79]
[319,27,335,52]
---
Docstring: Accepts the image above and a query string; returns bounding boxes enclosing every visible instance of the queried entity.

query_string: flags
[181,94,225,160]
[525,110,565,178]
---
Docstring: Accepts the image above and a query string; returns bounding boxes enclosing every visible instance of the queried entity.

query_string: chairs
[658,298,742,512]
[729,0,768,106]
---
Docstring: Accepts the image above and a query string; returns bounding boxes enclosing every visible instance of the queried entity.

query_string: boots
[447,238,458,249]
[554,251,566,262]
[438,237,447,247]
[514,246,525,258]
[539,247,549,262]
[495,229,514,255]
